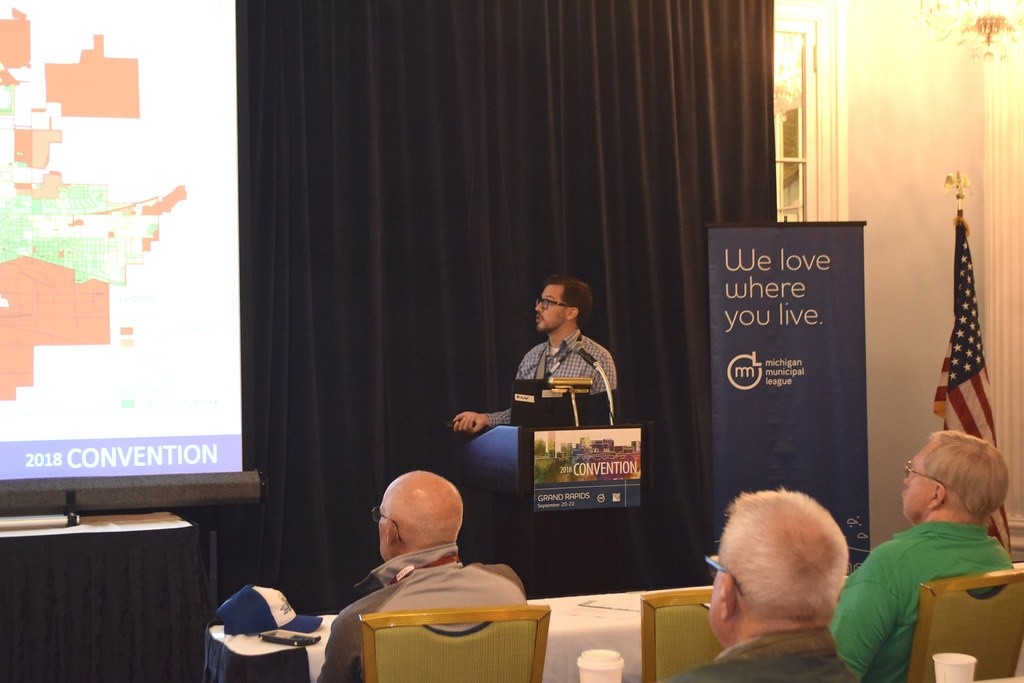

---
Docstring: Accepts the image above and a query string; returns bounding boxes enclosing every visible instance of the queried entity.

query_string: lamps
[921,0,1024,70]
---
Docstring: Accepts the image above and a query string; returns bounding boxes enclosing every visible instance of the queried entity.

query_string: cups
[574,648,625,683]
[931,651,979,682]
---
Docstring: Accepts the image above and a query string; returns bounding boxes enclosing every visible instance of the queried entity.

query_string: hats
[216,584,323,635]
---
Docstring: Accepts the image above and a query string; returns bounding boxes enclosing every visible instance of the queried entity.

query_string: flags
[932,218,1012,560]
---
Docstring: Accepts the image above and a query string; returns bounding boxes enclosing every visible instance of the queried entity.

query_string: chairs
[907,568,1024,683]
[640,588,724,683]
[359,606,550,683]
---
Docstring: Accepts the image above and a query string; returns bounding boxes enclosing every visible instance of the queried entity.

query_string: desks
[207,590,641,683]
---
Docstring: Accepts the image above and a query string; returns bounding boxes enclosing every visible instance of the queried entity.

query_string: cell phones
[259,630,321,647]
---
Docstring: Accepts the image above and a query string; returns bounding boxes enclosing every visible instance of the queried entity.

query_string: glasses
[905,460,945,504]
[535,298,571,310]
[704,554,743,598]
[372,506,402,543]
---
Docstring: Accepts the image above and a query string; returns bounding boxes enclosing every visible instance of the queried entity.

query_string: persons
[450,271,617,436]
[315,470,528,682]
[830,429,1015,683]
[658,484,861,683]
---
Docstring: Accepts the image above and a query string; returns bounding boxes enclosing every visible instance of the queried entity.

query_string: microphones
[575,343,600,372]
